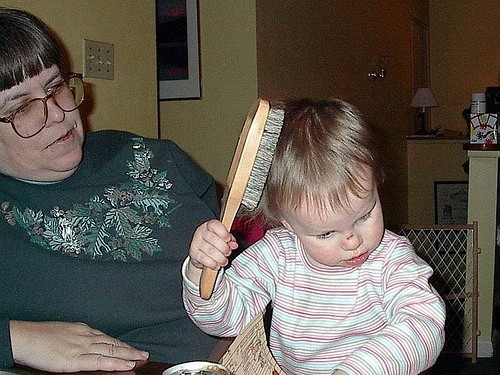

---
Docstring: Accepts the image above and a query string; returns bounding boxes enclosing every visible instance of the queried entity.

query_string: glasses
[0,71,84,138]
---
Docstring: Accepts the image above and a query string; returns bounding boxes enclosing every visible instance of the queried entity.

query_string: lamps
[404,87,439,138]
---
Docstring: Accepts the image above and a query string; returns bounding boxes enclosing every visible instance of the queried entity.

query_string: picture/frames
[155,0,201,101]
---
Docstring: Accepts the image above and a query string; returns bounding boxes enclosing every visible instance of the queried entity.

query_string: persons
[0,7,252,374]
[178,95,448,375]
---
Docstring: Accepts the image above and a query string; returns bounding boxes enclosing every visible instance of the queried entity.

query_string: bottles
[471,92,486,114]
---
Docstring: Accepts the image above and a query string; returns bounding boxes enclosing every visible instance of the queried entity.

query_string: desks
[0,360,174,374]
